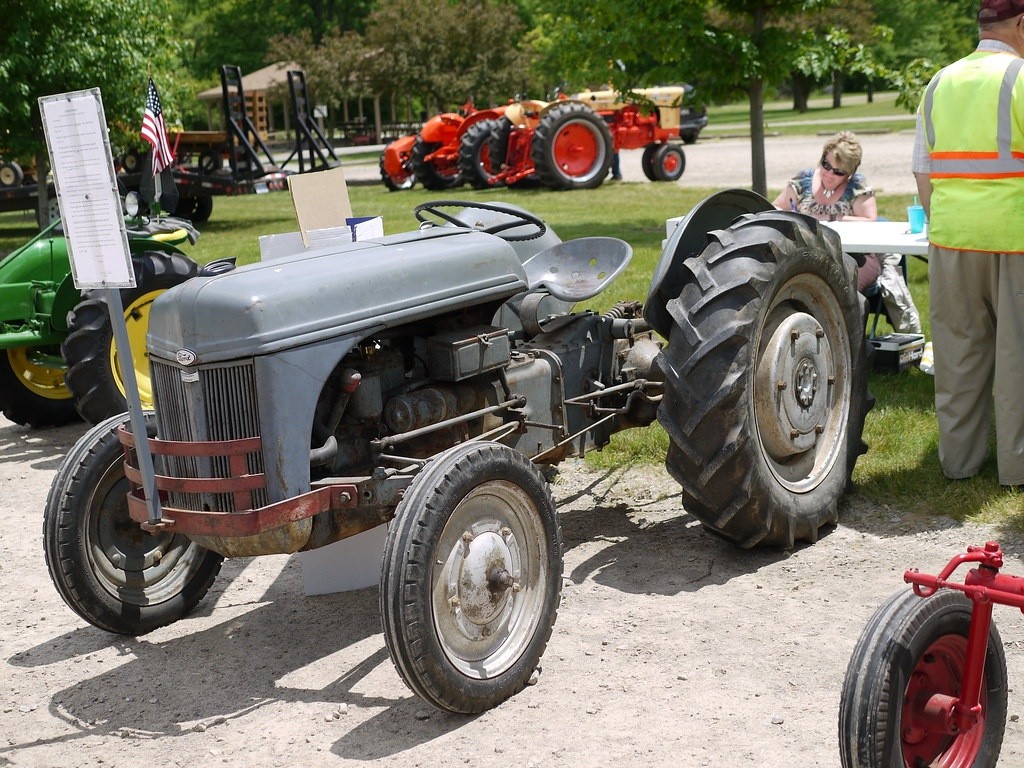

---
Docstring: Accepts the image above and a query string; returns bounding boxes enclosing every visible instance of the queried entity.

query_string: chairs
[867,218,909,339]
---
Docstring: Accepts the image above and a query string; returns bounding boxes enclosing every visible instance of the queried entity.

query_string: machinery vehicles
[837,540,1024,767]
[40,188,876,715]
[379,81,709,192]
[0,63,344,430]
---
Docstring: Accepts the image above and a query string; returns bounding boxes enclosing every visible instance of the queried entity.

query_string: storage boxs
[867,332,925,367]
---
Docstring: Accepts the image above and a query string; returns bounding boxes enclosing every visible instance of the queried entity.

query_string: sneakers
[604,169,609,178]
[611,174,621,180]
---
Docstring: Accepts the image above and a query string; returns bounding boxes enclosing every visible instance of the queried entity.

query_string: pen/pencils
[790,198,796,212]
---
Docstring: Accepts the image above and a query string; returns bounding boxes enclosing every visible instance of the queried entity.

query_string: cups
[907,206,924,233]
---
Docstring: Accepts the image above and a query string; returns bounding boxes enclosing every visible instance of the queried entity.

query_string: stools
[503,236,633,335]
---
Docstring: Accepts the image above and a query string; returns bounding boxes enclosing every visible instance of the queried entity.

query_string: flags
[141,78,176,180]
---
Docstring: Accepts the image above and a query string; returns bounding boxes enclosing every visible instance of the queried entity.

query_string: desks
[815,218,932,255]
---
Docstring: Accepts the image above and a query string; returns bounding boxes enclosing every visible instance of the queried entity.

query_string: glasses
[822,156,846,176]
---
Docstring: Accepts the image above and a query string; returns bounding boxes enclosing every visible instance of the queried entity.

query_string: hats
[523,93,528,96]
[977,0,1024,24]
[515,94,520,98]
[554,87,560,95]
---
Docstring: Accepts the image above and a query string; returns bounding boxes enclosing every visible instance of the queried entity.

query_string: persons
[599,83,622,180]
[910,1,1024,492]
[771,130,882,291]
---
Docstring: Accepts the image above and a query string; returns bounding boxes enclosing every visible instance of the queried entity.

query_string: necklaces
[821,181,841,198]
